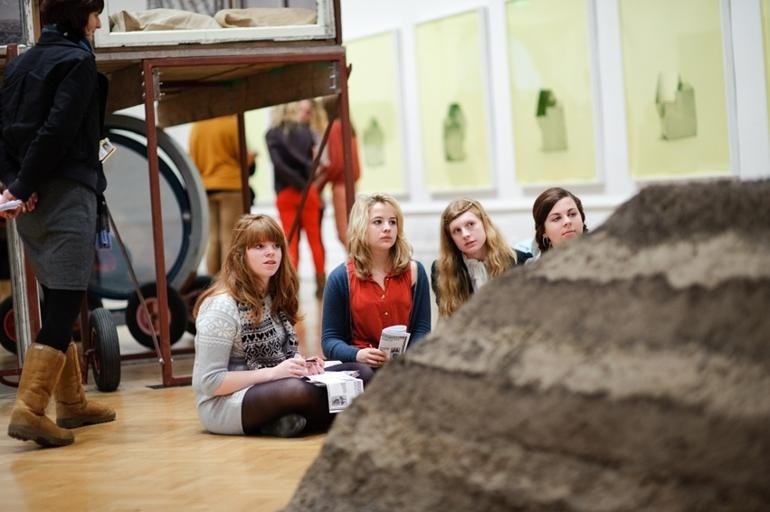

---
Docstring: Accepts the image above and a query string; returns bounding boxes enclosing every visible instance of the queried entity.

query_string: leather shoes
[245,412,307,437]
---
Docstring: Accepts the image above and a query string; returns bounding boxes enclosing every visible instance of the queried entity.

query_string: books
[300,369,364,413]
[378,325,411,360]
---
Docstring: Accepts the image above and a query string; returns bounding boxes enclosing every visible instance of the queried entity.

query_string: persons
[431,199,533,320]
[321,191,431,371]
[443,104,465,160]
[192,215,374,438]
[536,90,568,151]
[525,187,588,265]
[189,94,359,300]
[0,0,116,447]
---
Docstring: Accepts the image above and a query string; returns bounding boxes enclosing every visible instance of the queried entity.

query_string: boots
[8,342,115,446]
